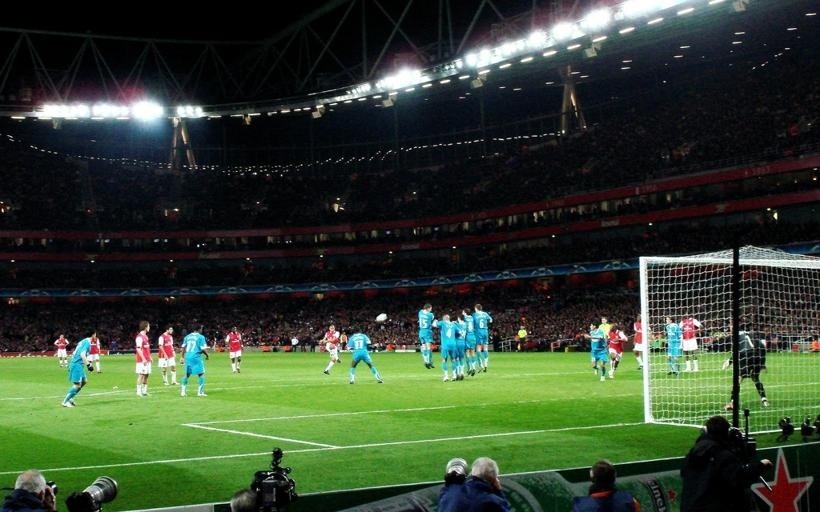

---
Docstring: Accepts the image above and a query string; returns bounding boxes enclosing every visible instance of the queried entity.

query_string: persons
[434,308,476,382]
[0,469,58,512]
[180,323,209,395]
[666,312,703,374]
[230,489,259,512]
[437,456,512,511]
[585,316,626,381]
[349,326,384,384]
[570,459,641,512]
[88,338,103,373]
[135,320,152,395]
[2,66,818,351]
[225,326,244,373]
[159,324,179,386]
[471,303,493,373]
[418,304,435,369]
[56,334,69,367]
[63,329,96,406]
[635,316,644,368]
[678,417,774,512]
[721,324,770,409]
[320,323,341,373]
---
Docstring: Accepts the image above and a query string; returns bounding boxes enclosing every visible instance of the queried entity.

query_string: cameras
[46,481,56,497]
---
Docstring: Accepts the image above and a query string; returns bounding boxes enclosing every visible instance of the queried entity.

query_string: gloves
[721,359,731,370]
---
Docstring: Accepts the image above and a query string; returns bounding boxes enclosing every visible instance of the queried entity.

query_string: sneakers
[163,379,181,387]
[96,371,103,374]
[336,359,342,363]
[197,393,208,397]
[137,393,152,398]
[667,368,700,377]
[600,375,606,382]
[377,379,384,383]
[61,400,77,407]
[323,368,330,375]
[594,364,600,375]
[762,398,770,408]
[723,399,734,411]
[232,367,241,374]
[636,363,644,371]
[608,369,616,379]
[422,361,488,383]
[349,378,355,384]
[180,393,186,396]
